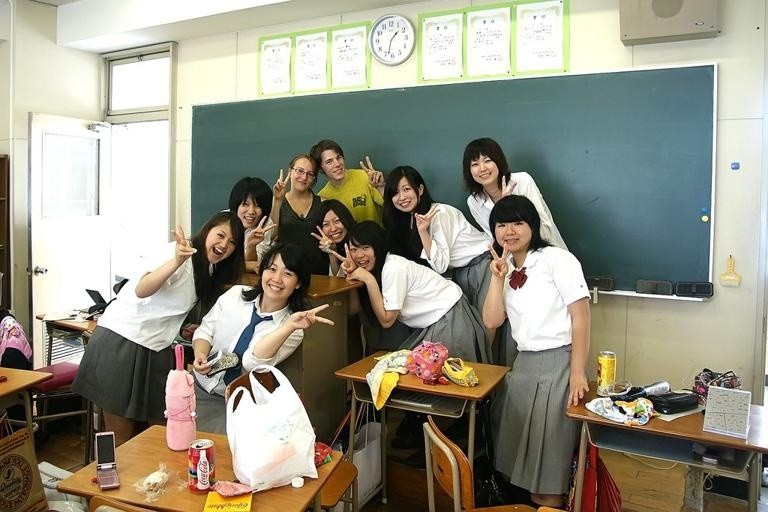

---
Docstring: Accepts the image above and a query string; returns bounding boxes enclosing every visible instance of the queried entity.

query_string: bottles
[195,450,210,490]
[620,379,669,401]
[163,345,197,452]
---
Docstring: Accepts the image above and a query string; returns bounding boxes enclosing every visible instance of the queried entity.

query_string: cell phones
[96,431,119,489]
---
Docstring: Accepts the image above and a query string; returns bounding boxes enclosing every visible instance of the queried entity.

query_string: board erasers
[584,276,615,291]
[636,279,713,297]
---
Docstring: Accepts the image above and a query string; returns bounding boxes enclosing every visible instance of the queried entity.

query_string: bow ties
[508,268,528,289]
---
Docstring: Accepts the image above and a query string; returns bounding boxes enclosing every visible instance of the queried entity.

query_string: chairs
[422,415,538,512]
[309,462,359,512]
[88,495,159,512]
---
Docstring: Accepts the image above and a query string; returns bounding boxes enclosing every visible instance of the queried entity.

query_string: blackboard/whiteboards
[191,62,718,302]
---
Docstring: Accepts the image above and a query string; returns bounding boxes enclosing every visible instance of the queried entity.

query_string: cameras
[200,350,222,367]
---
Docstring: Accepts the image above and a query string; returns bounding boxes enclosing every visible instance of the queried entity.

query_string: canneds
[187,439,217,495]
[596,350,617,397]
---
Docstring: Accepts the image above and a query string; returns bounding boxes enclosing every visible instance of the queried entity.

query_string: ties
[222,304,273,386]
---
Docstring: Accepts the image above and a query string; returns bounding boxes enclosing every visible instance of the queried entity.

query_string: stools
[27,361,90,467]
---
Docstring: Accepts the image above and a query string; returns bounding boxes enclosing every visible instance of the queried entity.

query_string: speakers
[619,0,717,46]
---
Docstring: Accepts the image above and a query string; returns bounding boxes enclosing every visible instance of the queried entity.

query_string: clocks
[368,12,417,67]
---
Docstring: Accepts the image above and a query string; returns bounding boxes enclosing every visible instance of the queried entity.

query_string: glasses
[291,165,317,180]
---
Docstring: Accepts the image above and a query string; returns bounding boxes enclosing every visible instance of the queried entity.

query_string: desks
[334,351,511,512]
[565,378,766,512]
[57,424,343,512]
[0,367,54,439]
[36,306,97,465]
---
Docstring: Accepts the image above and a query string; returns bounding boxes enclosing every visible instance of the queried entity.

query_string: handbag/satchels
[650,391,699,414]
[0,407,50,511]
[324,401,387,512]
[474,461,521,508]
[565,442,622,512]
[694,366,742,407]
